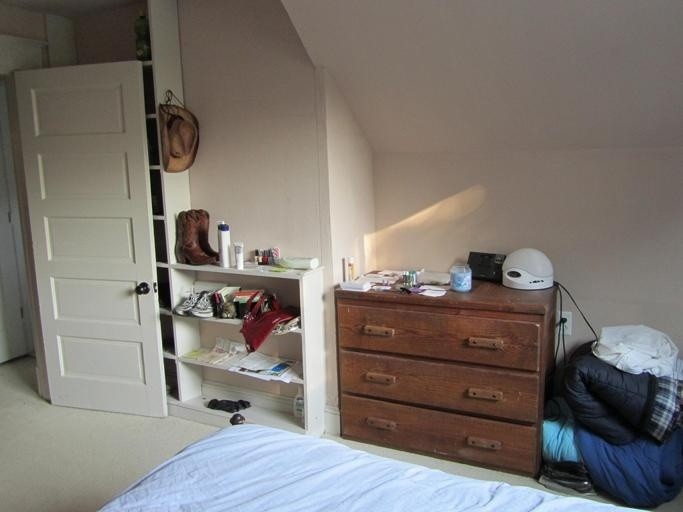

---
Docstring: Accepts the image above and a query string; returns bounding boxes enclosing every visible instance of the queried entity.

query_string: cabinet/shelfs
[168,258,327,439]
[42,0,203,419]
[335,271,557,479]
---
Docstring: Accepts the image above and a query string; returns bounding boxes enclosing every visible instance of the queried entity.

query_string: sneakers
[174,293,202,316]
[191,290,217,318]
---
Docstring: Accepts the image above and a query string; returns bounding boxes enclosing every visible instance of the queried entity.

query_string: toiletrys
[254,249,273,265]
[233,243,243,269]
[402,271,416,285]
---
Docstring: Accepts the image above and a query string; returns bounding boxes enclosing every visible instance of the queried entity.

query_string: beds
[94,424,647,512]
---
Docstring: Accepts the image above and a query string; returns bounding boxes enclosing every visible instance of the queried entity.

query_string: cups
[450,263,472,292]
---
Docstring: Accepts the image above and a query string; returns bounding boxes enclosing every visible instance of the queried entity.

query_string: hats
[159,104,199,173]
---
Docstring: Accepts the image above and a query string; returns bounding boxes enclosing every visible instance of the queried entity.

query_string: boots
[188,209,220,261]
[175,210,217,265]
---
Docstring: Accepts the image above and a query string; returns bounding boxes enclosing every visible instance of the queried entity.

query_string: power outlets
[557,311,572,337]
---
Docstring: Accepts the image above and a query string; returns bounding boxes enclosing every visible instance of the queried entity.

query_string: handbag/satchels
[240,291,300,353]
